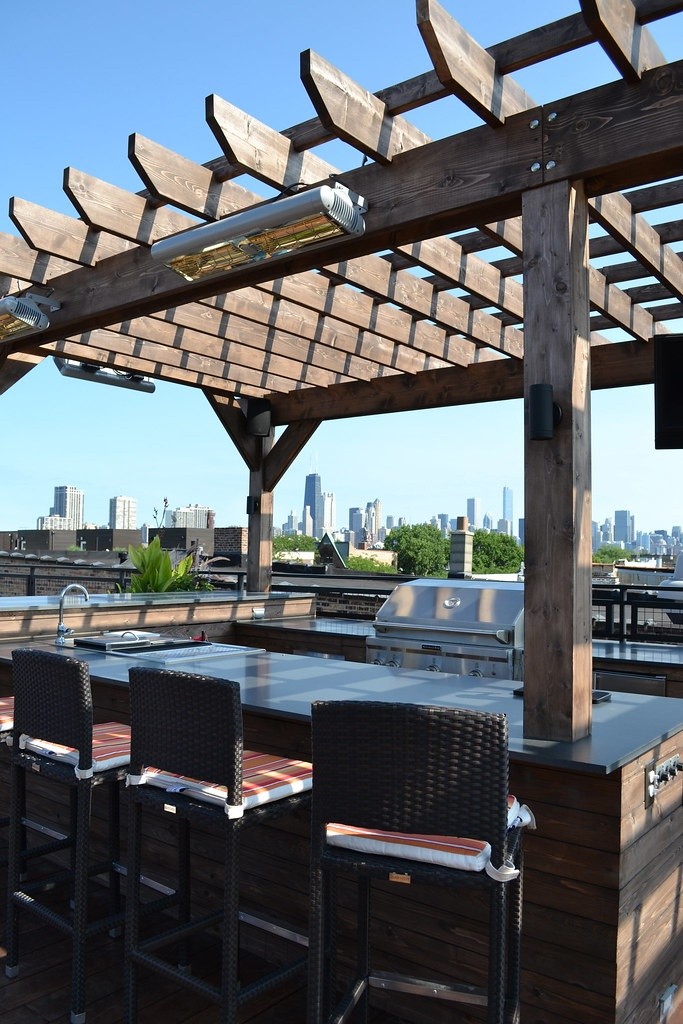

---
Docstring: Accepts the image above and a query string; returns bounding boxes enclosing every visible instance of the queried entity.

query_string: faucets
[54,581,91,644]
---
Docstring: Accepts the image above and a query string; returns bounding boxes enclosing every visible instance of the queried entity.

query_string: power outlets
[654,748,681,792]
[645,761,656,808]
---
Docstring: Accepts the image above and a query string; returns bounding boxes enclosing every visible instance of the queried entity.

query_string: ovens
[361,578,524,680]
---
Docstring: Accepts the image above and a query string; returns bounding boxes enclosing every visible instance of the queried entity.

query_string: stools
[6,648,187,1022]
[118,664,323,1024]
[309,701,521,1024]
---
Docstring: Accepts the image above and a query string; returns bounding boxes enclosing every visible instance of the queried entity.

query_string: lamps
[152,186,369,282]
[0,290,58,345]
